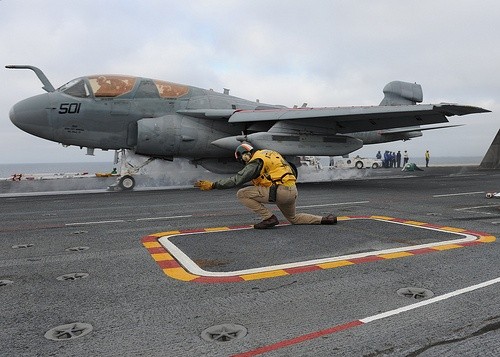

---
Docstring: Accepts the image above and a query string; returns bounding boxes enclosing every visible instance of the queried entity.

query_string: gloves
[194,180,214,191]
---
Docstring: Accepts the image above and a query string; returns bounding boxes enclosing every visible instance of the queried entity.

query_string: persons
[403,150,409,166]
[96,76,113,93]
[425,150,430,168]
[376,150,402,168]
[194,144,338,228]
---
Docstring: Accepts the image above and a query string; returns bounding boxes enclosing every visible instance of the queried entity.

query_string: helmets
[235,143,254,160]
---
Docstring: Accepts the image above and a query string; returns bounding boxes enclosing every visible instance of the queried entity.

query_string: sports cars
[335,156,383,169]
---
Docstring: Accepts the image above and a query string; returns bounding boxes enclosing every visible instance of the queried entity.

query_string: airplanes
[6,64,492,191]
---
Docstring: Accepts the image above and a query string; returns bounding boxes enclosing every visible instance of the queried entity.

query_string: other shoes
[254,215,280,229]
[321,213,337,224]
